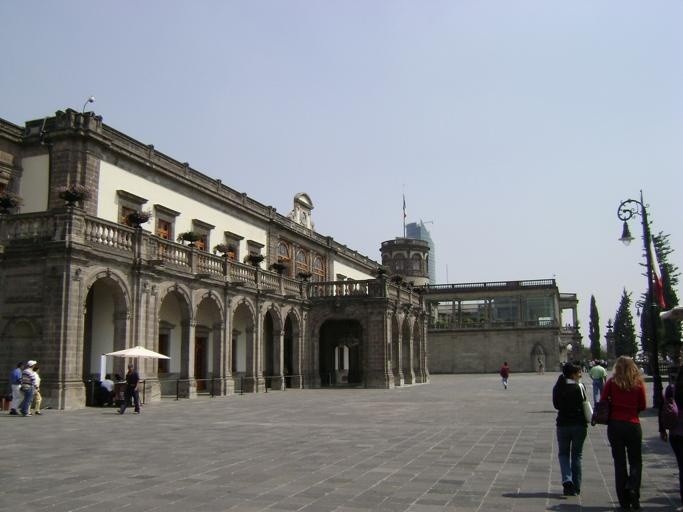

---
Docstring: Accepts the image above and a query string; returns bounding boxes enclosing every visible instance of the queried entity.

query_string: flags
[650,237,669,307]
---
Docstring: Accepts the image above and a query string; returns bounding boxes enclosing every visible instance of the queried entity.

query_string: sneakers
[10,408,42,417]
[622,490,640,512]
[118,411,140,414]
[563,481,580,496]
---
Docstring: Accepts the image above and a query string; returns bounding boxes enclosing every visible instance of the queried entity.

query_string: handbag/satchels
[661,399,678,424]
[583,402,593,423]
[593,402,610,424]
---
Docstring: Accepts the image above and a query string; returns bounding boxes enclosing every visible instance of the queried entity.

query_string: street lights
[616,196,666,408]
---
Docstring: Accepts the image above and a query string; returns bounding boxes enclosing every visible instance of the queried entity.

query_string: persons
[552,360,593,496]
[28,366,43,415]
[500,362,510,389]
[117,364,140,415]
[557,357,608,373]
[659,367,683,499]
[8,362,25,415]
[101,374,116,406]
[537,357,544,375]
[589,359,608,407]
[21,360,36,416]
[600,355,646,510]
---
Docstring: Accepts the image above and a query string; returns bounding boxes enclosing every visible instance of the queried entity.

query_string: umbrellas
[104,345,171,373]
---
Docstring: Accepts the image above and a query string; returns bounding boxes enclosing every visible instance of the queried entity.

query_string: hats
[28,360,37,366]
